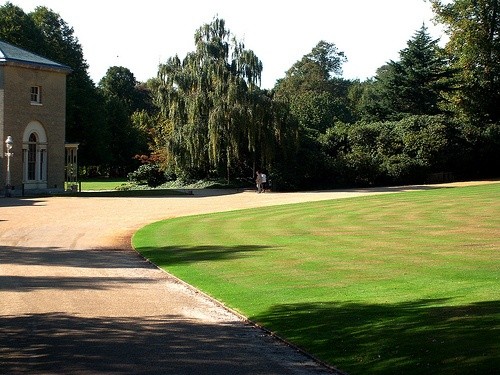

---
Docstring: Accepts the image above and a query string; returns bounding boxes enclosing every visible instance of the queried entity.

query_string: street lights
[5,136,13,197]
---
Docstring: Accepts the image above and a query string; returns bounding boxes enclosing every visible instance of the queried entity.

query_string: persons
[268,178,273,193]
[255,171,262,194]
[260,171,266,193]
